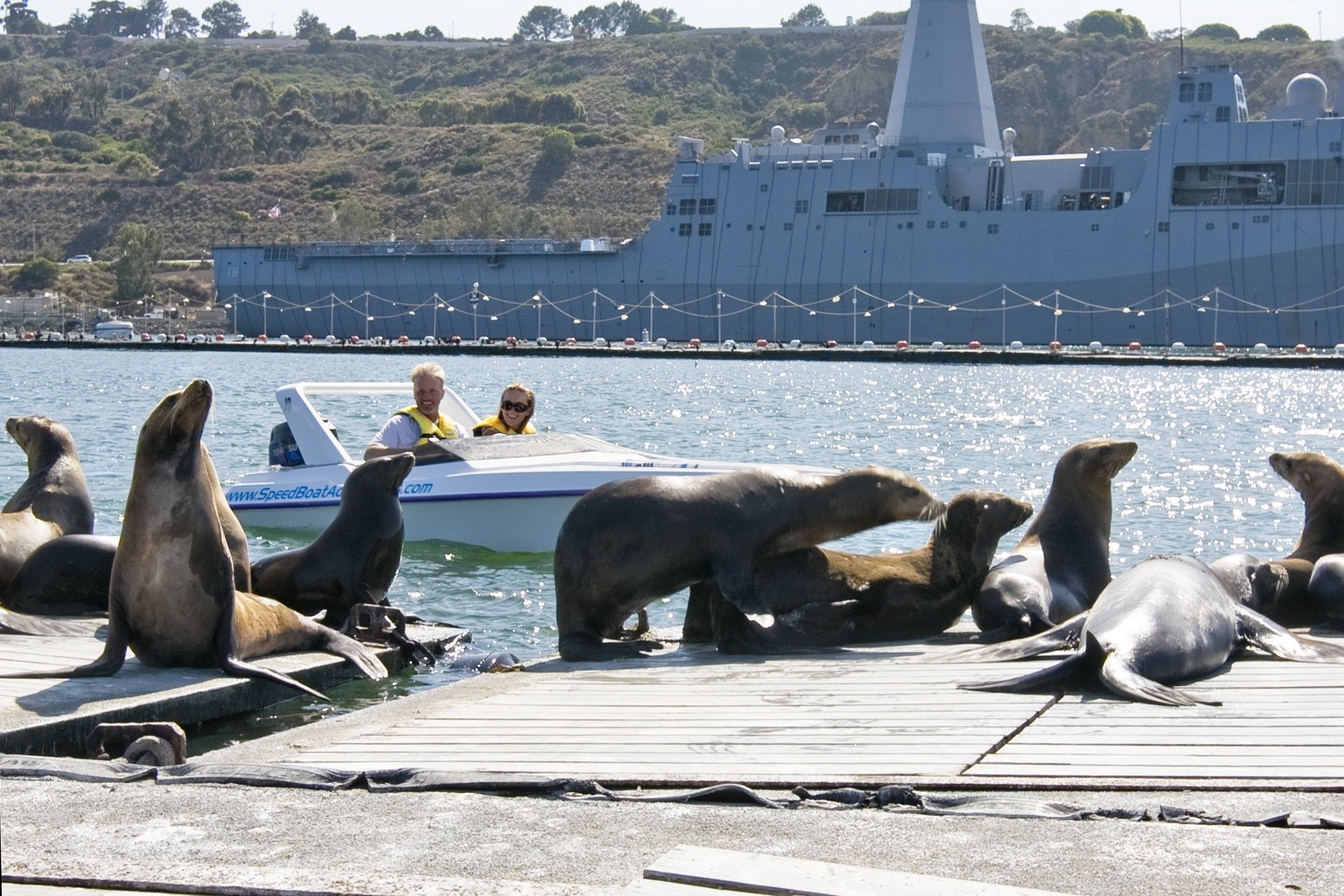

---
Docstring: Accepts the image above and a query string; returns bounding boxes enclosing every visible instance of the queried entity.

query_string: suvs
[66,255,92,264]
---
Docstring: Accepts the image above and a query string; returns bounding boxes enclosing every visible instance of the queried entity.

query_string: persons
[363,362,470,460]
[473,384,538,437]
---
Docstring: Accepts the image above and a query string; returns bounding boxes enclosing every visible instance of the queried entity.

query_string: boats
[218,3,1344,351]
[226,380,847,556]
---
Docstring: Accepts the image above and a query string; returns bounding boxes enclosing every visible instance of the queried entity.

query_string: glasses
[499,400,530,413]
